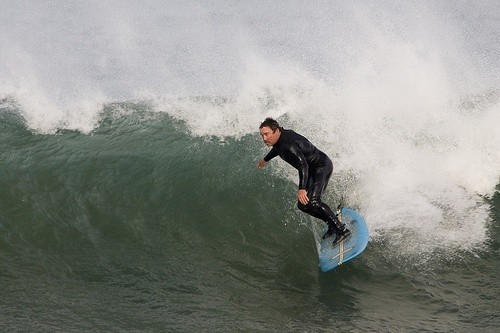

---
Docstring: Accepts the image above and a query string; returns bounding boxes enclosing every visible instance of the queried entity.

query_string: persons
[255,117,350,246]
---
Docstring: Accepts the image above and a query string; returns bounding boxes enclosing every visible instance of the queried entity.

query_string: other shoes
[323,226,334,239]
[333,229,350,246]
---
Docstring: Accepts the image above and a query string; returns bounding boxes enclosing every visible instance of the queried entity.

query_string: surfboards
[319,208,370,271]
